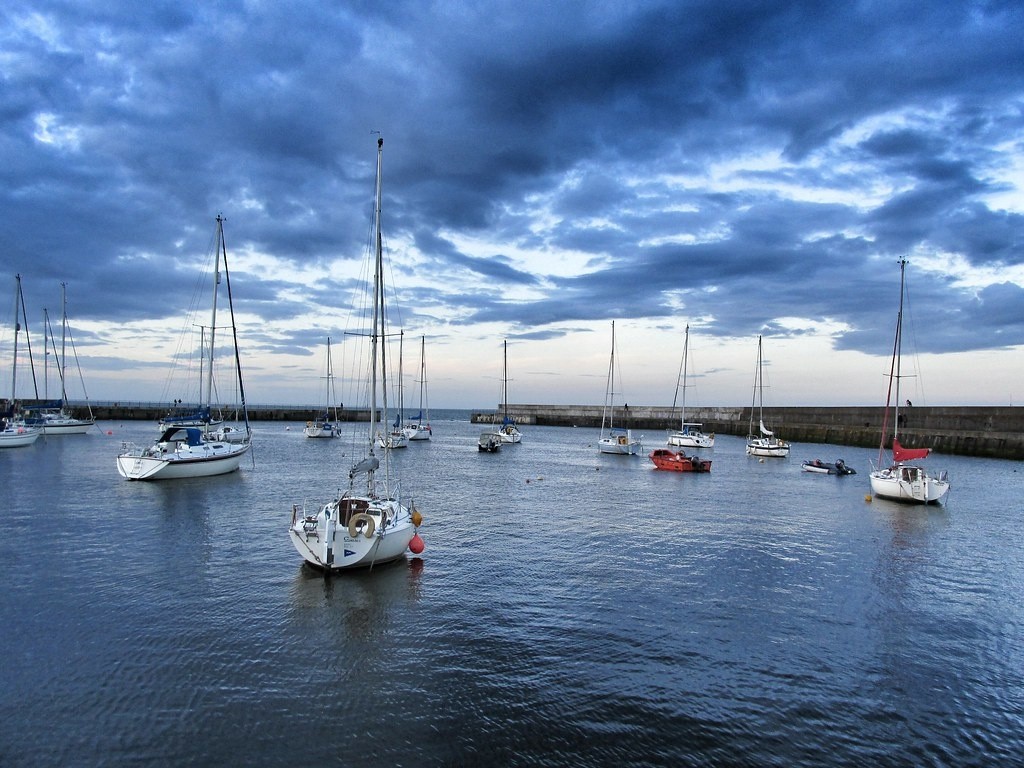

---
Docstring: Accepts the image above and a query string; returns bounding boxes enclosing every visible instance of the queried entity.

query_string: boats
[801,458,856,474]
[479,432,502,455]
[649,449,712,472]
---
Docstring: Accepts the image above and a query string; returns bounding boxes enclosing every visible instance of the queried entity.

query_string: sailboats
[377,330,410,449]
[868,255,950,502]
[744,336,790,458]
[666,323,716,449]
[13,306,78,430]
[158,324,221,433]
[0,274,45,448]
[402,335,432,440]
[303,337,342,438]
[193,326,252,444]
[23,279,95,436]
[498,340,523,444]
[597,321,644,456]
[286,130,423,568]
[116,213,252,480]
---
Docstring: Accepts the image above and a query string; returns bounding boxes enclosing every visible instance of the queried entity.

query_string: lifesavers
[349,513,375,538]
[709,434,714,439]
[621,438,627,445]
[307,421,313,427]
[778,440,784,446]
